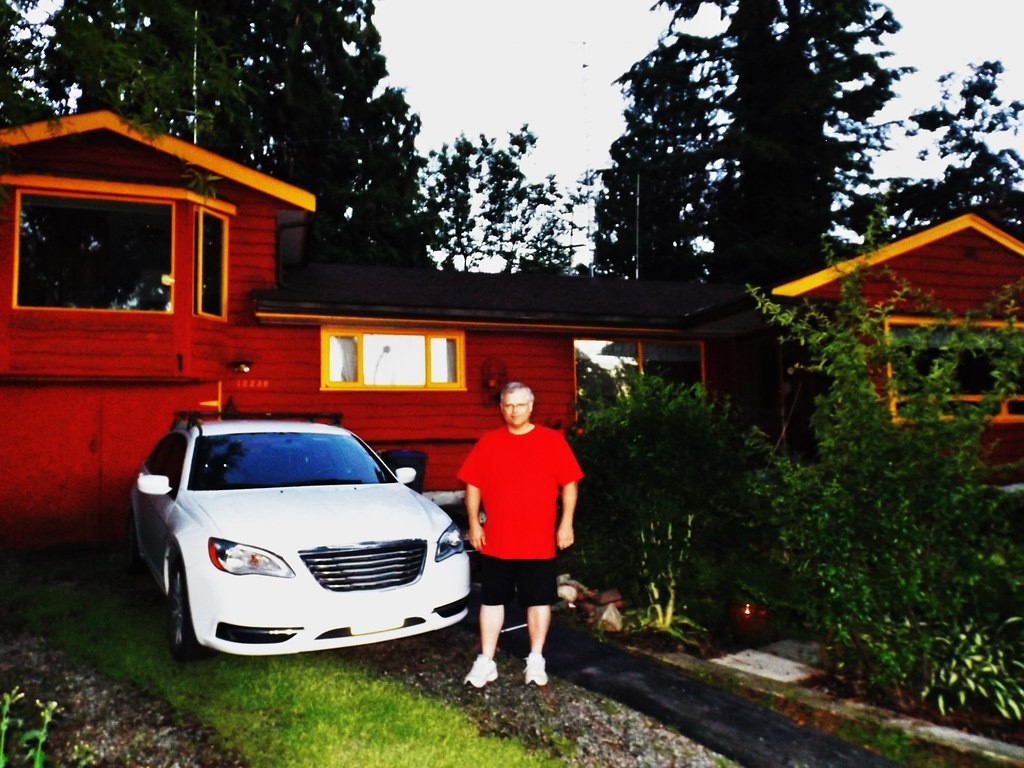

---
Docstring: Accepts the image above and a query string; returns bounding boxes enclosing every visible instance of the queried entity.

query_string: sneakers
[463,654,499,689]
[523,654,548,686]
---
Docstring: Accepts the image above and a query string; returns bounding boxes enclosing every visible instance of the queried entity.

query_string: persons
[456,381,585,689]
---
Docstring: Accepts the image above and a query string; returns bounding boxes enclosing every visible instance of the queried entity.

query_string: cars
[121,406,475,663]
